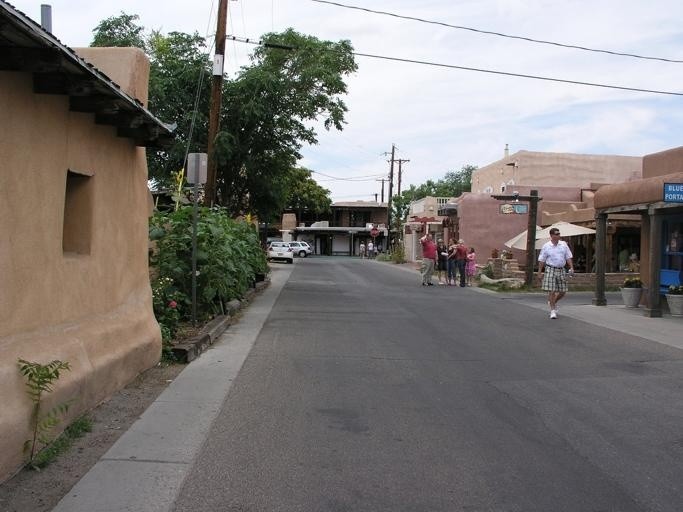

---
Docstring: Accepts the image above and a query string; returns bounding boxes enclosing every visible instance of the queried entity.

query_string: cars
[266,241,293,264]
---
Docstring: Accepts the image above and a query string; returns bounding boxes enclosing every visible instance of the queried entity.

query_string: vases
[664,292,683,316]
[619,287,642,309]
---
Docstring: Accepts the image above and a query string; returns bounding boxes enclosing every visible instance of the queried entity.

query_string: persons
[421,233,475,287]
[576,244,629,272]
[360,240,382,259]
[538,228,574,319]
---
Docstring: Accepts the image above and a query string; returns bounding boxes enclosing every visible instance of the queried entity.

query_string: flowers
[623,276,641,288]
[669,283,683,293]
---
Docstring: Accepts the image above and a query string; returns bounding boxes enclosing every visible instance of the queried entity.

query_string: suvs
[288,241,312,258]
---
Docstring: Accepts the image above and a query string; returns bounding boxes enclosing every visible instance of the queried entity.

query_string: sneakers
[551,310,558,318]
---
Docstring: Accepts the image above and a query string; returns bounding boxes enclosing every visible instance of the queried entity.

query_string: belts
[546,264,563,269]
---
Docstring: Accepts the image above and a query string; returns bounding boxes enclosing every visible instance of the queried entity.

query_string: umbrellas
[536,221,596,237]
[504,225,568,250]
